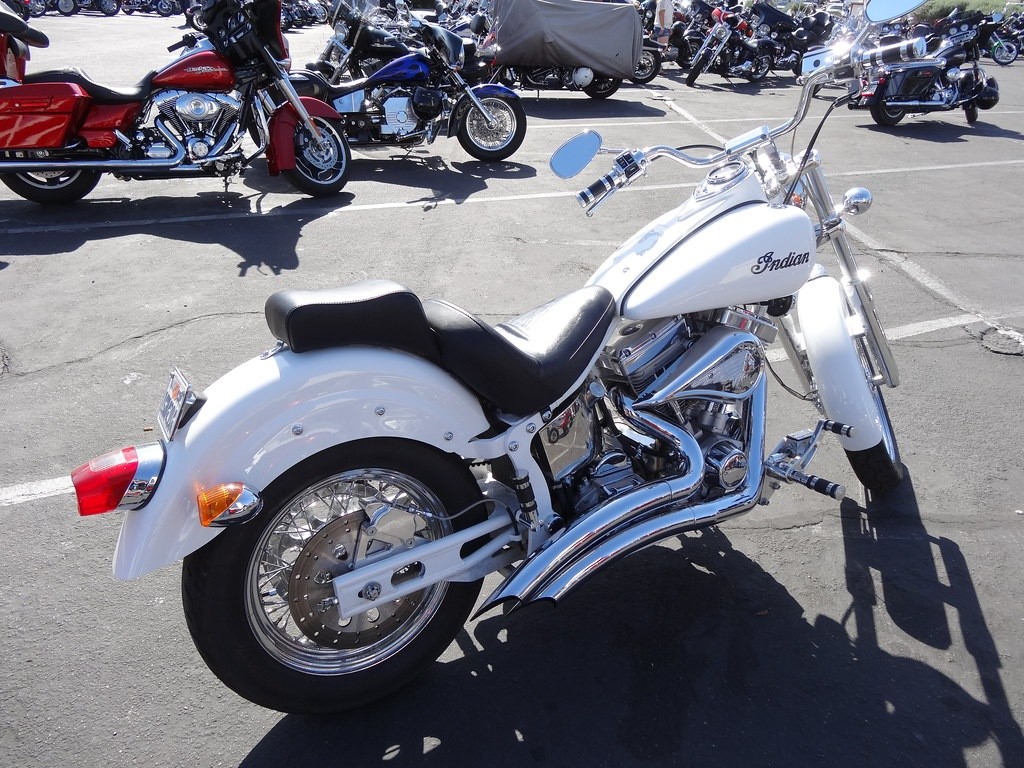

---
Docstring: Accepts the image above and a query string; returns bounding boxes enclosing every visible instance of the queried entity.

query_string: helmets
[975,74,1000,109]
[672,21,686,35]
[410,84,443,122]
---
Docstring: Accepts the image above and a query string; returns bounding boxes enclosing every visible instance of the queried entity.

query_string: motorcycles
[3,0,208,31]
[248,19,526,164]
[627,0,1024,127]
[281,1,623,99]
[1,0,353,204]
[72,0,925,717]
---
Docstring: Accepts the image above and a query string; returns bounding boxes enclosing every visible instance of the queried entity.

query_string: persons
[654,0,675,75]
